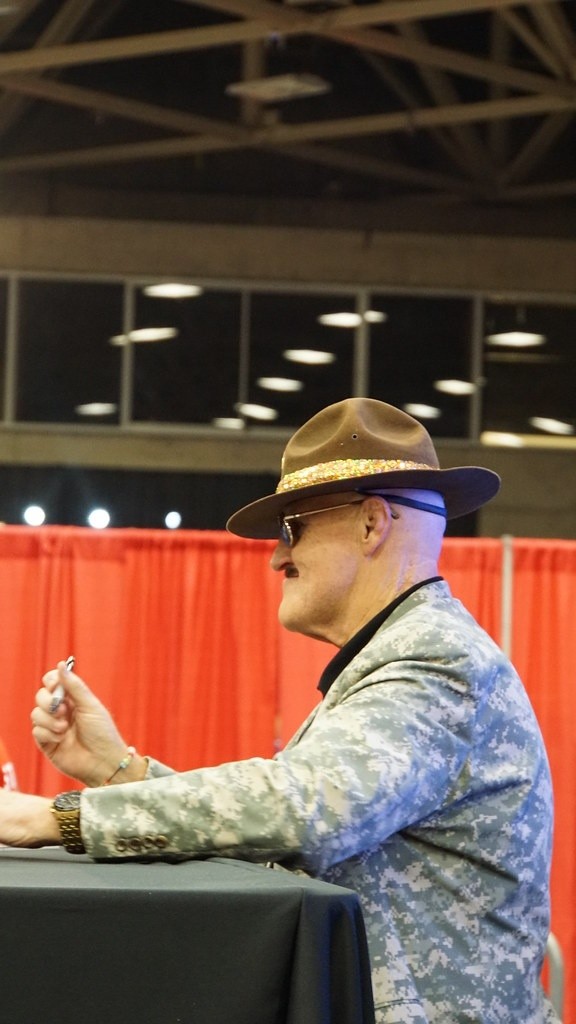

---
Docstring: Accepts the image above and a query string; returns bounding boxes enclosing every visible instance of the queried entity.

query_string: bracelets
[98,745,136,787]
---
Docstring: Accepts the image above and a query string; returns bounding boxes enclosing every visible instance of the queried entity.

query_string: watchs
[48,790,87,854]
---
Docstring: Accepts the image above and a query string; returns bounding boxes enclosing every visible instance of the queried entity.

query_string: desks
[0,843,376,1024]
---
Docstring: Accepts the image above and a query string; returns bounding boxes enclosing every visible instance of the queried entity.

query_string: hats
[228,395,502,543]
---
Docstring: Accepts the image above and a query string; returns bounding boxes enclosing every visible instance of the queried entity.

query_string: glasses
[278,491,403,549]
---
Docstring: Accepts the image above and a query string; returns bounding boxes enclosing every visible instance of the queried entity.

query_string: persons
[1,398,565,1023]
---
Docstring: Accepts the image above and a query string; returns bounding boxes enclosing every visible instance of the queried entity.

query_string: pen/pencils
[48,655,75,711]
